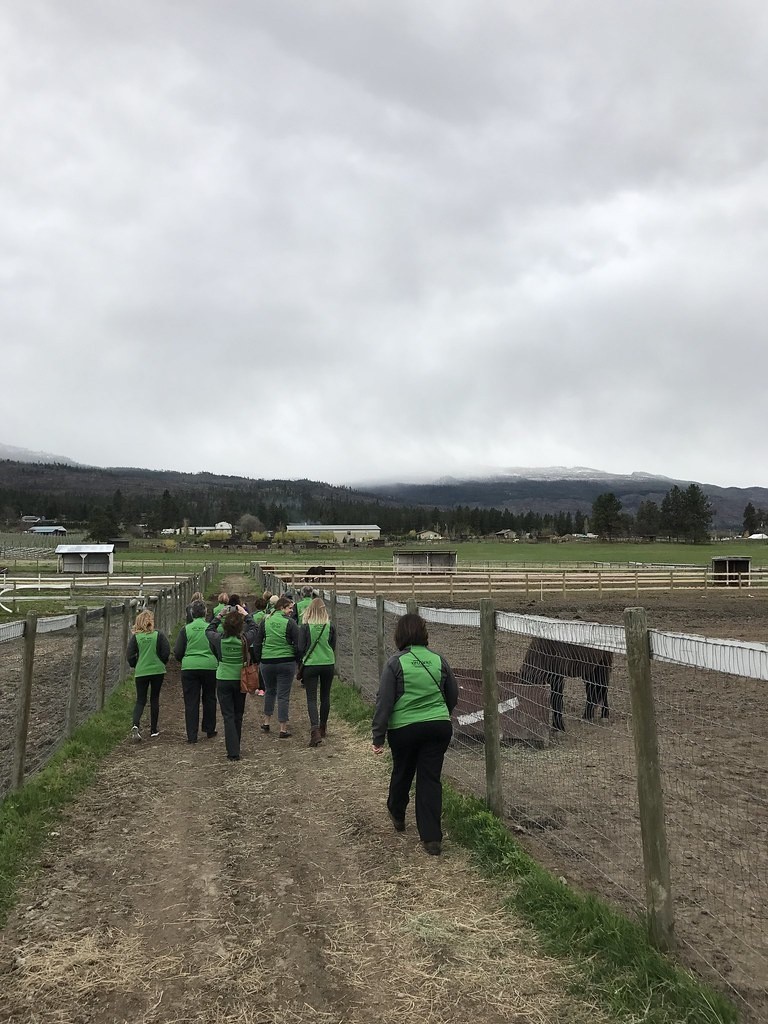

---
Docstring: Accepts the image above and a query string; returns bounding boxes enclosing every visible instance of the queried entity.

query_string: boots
[309,728,322,747]
[320,725,327,738]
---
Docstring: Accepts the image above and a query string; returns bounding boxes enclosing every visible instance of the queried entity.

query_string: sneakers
[131,725,142,743]
[150,729,159,737]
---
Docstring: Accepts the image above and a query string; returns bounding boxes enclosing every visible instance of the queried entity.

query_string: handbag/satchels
[240,635,260,693]
[297,664,304,680]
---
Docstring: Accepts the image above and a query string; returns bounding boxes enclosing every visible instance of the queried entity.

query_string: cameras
[229,605,238,612]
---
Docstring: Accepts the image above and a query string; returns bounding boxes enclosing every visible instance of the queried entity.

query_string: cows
[300,566,336,583]
[520,621,615,738]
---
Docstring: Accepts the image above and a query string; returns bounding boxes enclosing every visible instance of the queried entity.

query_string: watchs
[218,613,223,617]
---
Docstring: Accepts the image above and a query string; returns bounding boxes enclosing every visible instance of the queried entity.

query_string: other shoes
[227,755,240,761]
[279,731,291,738]
[207,731,217,738]
[388,811,405,831]
[423,841,441,855]
[261,724,269,730]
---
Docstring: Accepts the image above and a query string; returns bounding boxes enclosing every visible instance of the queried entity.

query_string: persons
[126,611,170,741]
[174,585,337,761]
[373,613,458,856]
